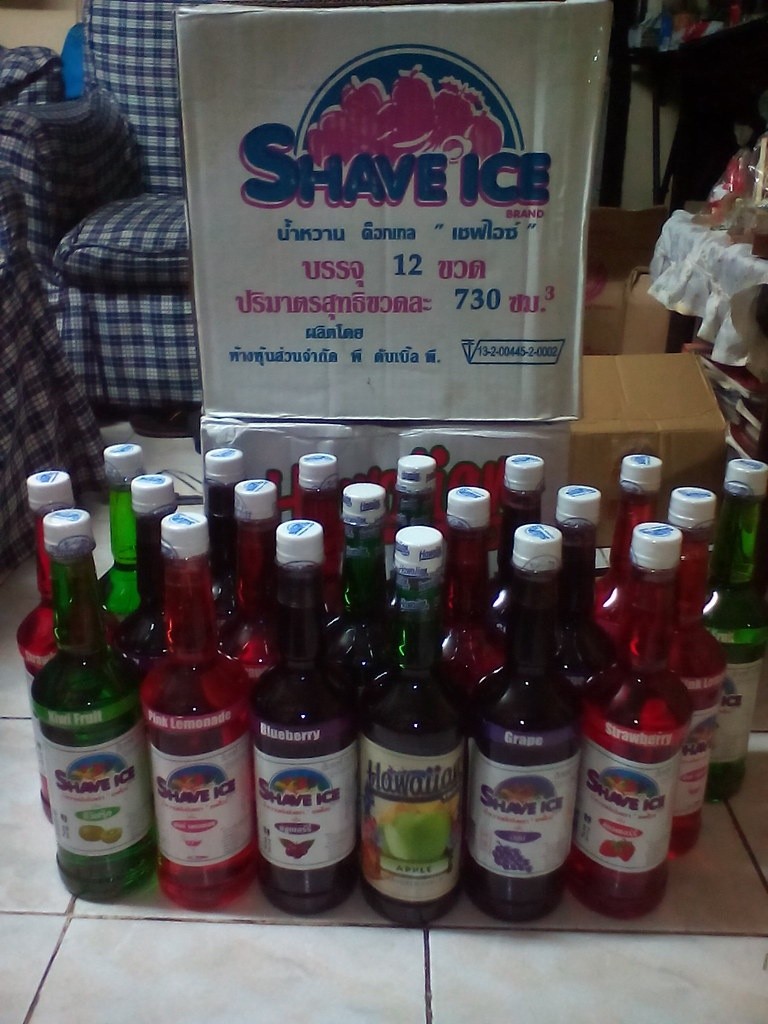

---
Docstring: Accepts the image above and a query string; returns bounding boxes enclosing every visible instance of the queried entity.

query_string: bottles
[17,443,768,919]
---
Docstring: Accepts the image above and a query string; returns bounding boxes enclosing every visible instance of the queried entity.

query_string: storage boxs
[201,349,730,578]
[173,1,679,424]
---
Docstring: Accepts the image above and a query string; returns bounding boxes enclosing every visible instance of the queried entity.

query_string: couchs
[0,0,209,576]
